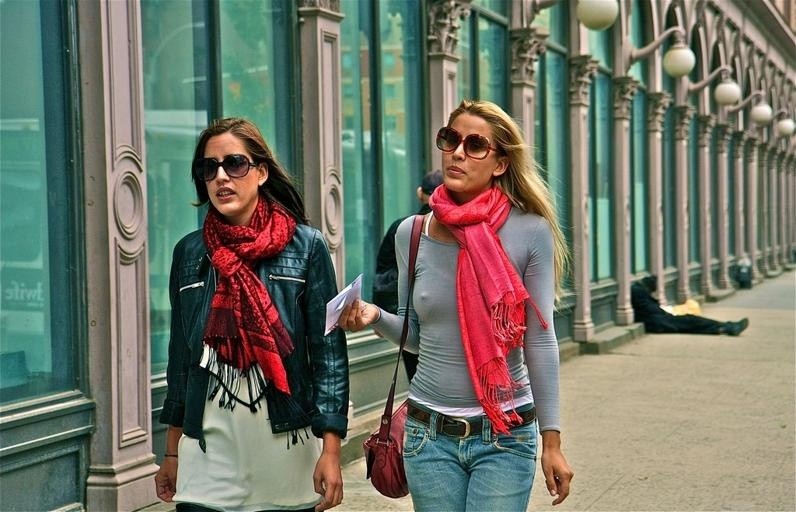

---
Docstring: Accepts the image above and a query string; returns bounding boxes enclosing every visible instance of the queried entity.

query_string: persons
[154,115,351,512]
[337,98,575,511]
[631,274,749,336]
[371,169,444,385]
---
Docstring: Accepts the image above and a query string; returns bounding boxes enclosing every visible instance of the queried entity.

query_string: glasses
[436,128,503,159]
[192,154,256,181]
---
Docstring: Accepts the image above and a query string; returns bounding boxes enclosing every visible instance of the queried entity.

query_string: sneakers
[727,319,749,335]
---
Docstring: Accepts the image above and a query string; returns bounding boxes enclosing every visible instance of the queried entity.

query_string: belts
[406,404,535,436]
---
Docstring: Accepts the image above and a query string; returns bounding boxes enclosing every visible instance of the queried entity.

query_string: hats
[643,275,657,292]
[421,170,445,195]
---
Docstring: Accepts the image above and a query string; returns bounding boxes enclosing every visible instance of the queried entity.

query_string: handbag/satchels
[372,271,397,316]
[364,400,409,498]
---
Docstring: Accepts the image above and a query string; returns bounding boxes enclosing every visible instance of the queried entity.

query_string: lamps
[755,106,795,136]
[687,66,740,106]
[631,26,697,77]
[727,89,772,124]
[535,0,618,31]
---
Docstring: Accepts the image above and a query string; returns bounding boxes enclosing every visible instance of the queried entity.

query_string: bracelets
[162,452,178,459]
